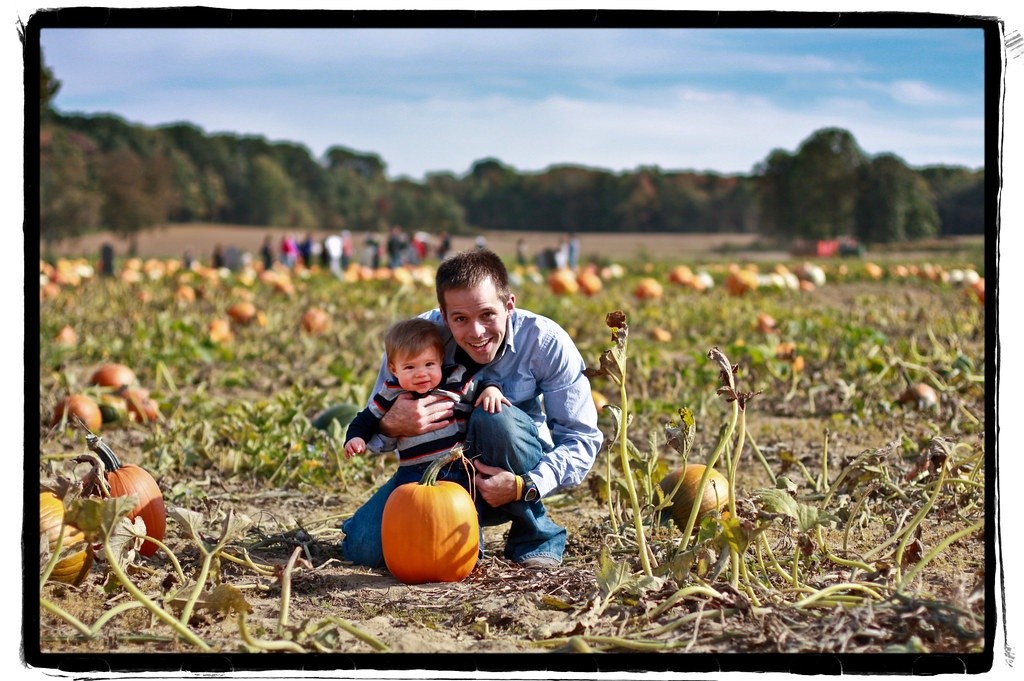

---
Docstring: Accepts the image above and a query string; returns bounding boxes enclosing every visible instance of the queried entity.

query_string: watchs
[521,474,538,502]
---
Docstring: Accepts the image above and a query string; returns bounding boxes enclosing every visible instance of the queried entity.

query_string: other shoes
[520,556,561,574]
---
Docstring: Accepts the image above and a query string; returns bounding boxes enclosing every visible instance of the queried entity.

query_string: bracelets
[516,476,522,500]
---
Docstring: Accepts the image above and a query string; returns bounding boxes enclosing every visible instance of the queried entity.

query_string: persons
[261,227,451,271]
[344,320,512,567]
[516,238,528,266]
[554,233,579,269]
[341,251,603,566]
[212,242,227,268]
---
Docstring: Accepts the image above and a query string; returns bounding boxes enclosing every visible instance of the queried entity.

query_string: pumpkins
[39,257,985,439]
[40,491,92,589]
[650,464,731,534]
[76,433,165,557]
[382,445,480,584]
